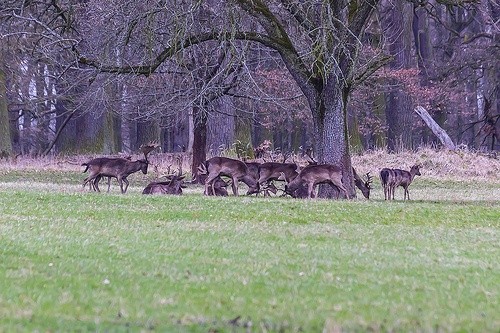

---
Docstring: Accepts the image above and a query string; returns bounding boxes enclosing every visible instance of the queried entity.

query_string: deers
[352,166,373,200]
[285,163,350,200]
[380,165,421,201]
[81,153,297,197]
